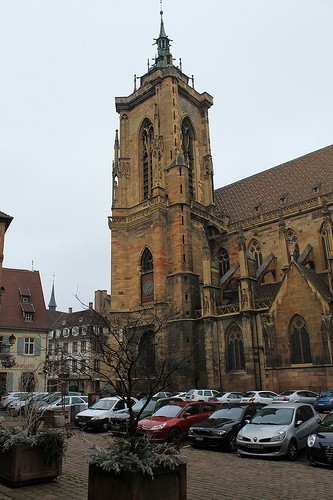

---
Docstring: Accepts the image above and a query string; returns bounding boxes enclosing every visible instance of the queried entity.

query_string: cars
[75,397,333,471]
[152,388,333,413]
[1,390,91,419]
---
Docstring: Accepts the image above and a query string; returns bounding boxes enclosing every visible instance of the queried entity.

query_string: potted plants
[0,398,73,488]
[74,294,202,500]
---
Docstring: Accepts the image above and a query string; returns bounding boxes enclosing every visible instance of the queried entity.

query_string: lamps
[0,334,16,350]
